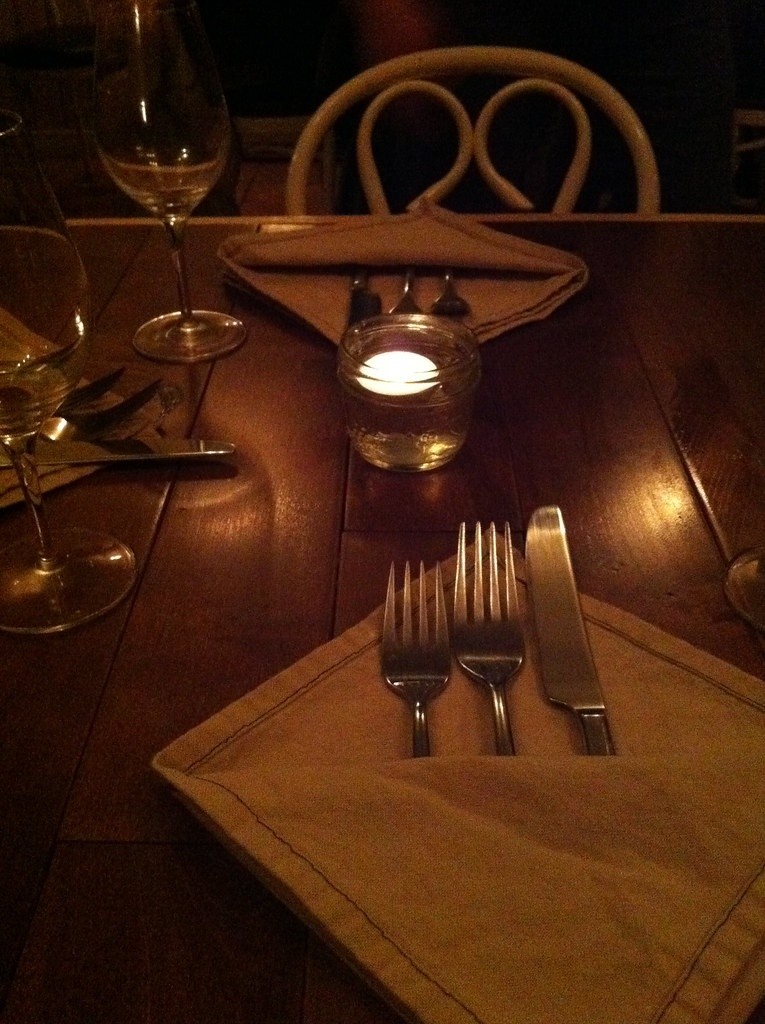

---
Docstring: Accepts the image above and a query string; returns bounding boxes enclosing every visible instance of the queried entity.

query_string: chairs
[285,44,662,216]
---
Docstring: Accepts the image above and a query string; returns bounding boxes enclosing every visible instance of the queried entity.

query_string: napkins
[214,195,590,347]
[153,528,765,1024]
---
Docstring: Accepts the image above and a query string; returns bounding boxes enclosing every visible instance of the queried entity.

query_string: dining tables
[0,216,765,1024]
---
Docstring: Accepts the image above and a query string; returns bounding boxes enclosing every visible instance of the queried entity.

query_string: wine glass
[724,546,765,629]
[86,0,245,362]
[0,108,134,633]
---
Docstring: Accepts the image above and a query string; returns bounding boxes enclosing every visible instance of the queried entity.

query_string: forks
[453,519,527,756]
[52,366,126,413]
[81,378,166,436]
[378,559,453,757]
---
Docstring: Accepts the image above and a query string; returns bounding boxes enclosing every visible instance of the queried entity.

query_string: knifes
[348,268,382,327]
[526,505,618,754]
[0,438,237,468]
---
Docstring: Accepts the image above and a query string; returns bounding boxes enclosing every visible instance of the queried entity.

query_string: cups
[337,314,479,472]
